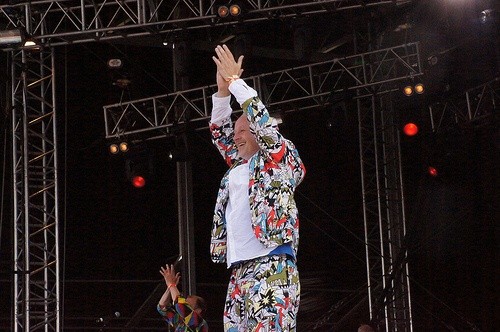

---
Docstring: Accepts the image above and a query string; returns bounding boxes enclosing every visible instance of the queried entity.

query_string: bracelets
[226,74,240,86]
[168,283,176,287]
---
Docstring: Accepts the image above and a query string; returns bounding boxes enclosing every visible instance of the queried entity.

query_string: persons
[157,264,208,332]
[209,43,306,332]
